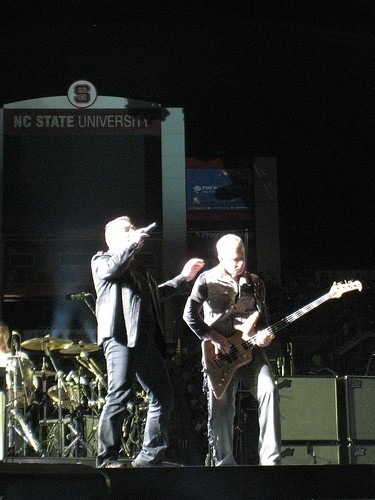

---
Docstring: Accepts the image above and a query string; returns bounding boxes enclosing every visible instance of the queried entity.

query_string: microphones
[66,292,91,301]
[143,222,158,234]
[11,331,17,356]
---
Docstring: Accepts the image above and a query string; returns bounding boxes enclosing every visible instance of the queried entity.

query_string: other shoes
[101,461,127,468]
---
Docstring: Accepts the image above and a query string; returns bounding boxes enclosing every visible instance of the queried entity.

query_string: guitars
[201,279,362,400]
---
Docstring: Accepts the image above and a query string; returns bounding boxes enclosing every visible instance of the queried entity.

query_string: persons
[182,233,281,465]
[0,321,37,455]
[91,216,205,468]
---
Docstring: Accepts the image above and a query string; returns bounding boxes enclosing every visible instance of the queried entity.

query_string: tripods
[4,340,111,458]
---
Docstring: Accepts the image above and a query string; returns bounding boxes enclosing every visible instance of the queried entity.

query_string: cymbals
[31,370,56,376]
[59,343,101,353]
[21,337,74,350]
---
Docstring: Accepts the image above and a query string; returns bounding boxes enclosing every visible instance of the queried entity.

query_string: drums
[49,383,82,410]
[0,357,32,391]
